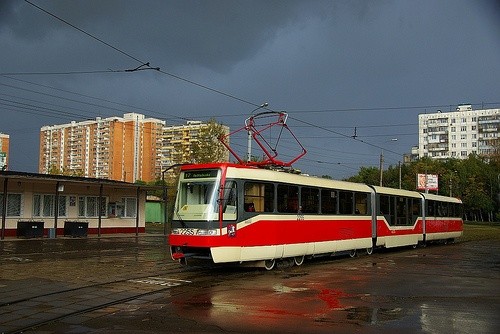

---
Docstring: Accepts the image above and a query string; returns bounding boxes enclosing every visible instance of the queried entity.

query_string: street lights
[246,102,270,162]
[379,138,397,187]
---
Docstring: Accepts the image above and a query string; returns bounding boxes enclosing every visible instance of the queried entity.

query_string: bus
[163,162,463,270]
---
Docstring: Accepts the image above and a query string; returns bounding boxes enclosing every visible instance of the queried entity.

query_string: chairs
[243,202,255,212]
